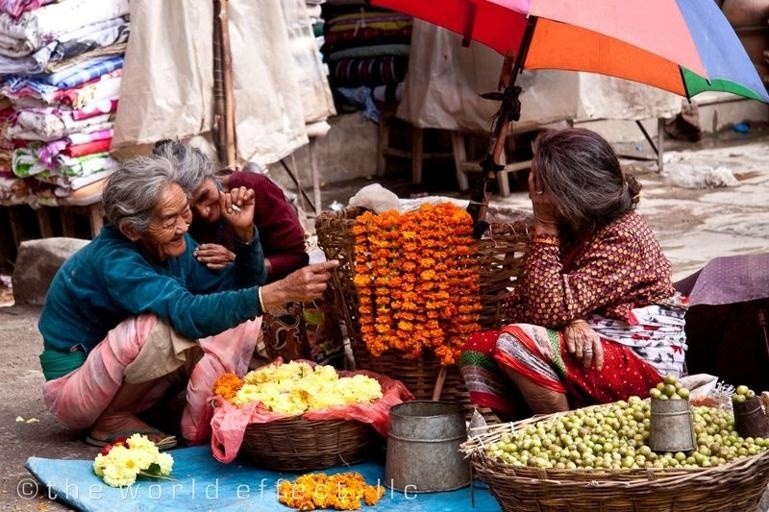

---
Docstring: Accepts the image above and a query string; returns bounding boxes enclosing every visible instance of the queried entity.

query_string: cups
[733,396,769,440]
[649,396,697,453]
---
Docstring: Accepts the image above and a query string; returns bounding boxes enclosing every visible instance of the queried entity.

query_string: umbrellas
[368,0,769,239]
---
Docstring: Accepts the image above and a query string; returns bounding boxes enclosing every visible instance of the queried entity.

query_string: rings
[535,188,545,198]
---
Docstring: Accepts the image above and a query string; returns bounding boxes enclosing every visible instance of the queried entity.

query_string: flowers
[278,472,386,512]
[93,432,176,487]
[211,201,481,417]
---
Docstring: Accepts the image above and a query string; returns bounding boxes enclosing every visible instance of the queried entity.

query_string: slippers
[83,423,178,453]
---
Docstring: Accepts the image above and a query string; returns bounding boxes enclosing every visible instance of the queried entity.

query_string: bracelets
[258,285,266,319]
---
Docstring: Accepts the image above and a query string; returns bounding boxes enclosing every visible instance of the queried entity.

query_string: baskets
[315,198,537,421]
[456,388,769,512]
[230,369,389,476]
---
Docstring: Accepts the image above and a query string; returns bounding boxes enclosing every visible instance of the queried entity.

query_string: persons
[36,155,339,456]
[154,136,310,285]
[450,125,692,422]
[230,201,245,214]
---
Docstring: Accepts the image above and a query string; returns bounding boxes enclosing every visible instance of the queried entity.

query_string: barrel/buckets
[386,399,472,495]
